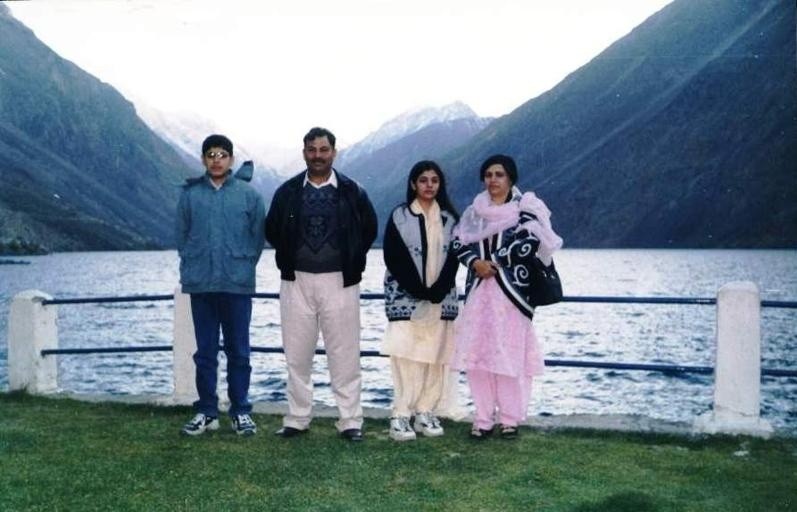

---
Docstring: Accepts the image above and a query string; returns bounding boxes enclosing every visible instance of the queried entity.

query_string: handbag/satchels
[527,259,563,307]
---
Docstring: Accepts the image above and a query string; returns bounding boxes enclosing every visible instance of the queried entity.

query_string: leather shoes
[340,428,362,441]
[274,427,309,438]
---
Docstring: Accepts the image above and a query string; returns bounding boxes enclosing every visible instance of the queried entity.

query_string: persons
[176,135,267,438]
[451,154,564,441]
[378,161,461,441]
[266,128,378,442]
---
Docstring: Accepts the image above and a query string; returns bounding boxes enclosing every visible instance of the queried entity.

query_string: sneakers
[500,427,518,440]
[470,427,493,440]
[231,413,257,437]
[388,417,416,442]
[413,414,443,437]
[180,413,220,438]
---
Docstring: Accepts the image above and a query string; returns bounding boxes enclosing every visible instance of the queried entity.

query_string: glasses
[203,151,229,161]
